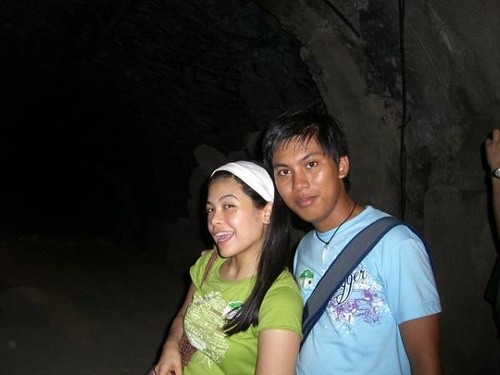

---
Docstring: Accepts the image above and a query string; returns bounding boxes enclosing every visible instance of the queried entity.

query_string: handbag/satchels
[146,249,219,375]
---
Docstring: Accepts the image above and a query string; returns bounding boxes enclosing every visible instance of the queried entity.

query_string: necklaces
[314,201,356,263]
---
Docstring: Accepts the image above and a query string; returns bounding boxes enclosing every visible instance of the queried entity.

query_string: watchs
[491,167,500,179]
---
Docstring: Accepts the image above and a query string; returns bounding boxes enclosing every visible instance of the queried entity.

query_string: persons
[260,104,444,375]
[484,129,500,330]
[146,159,303,375]
